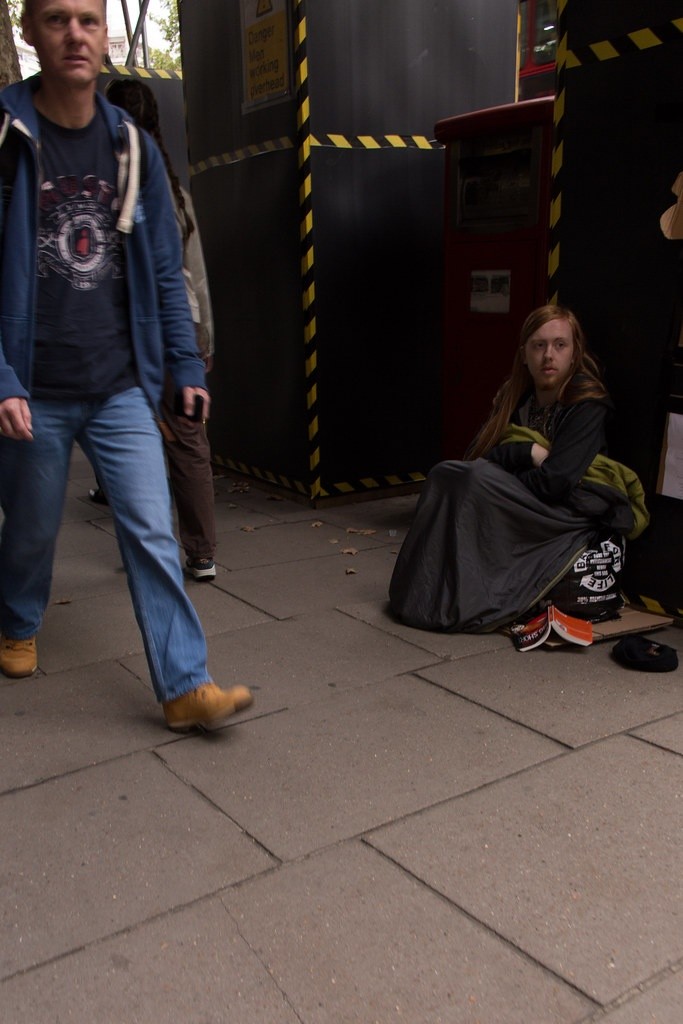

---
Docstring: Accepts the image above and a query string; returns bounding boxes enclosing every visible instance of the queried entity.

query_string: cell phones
[172,391,204,423]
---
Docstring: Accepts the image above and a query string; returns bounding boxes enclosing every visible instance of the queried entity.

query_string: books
[515,604,593,653]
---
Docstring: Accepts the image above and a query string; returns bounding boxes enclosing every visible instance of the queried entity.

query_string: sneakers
[0,634,37,678]
[162,682,254,728]
[186,556,217,578]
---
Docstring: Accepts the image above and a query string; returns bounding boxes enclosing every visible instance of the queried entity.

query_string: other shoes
[88,488,110,505]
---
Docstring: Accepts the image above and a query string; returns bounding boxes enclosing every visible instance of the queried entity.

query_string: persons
[0,0,255,735]
[421,306,634,625]
[88,76,216,578]
